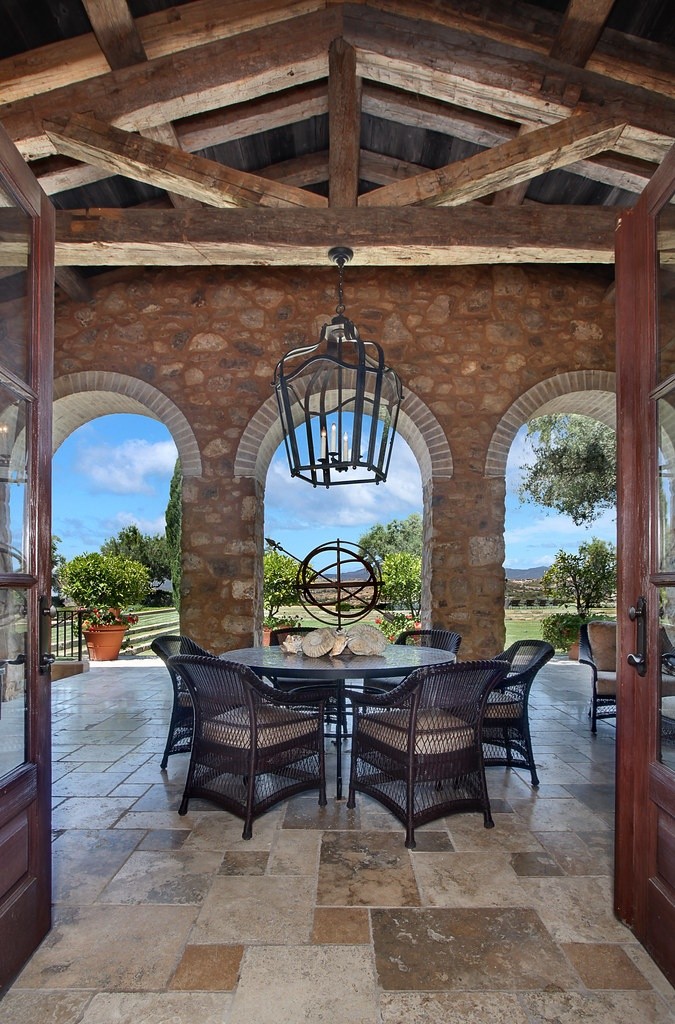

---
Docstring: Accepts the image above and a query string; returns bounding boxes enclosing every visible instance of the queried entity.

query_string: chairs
[341,660,511,849]
[482,639,555,786]
[662,695,675,743]
[151,635,220,770]
[362,629,462,713]
[165,654,327,841]
[269,627,348,740]
[578,621,675,733]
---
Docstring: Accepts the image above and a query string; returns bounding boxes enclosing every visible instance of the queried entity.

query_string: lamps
[269,246,405,490]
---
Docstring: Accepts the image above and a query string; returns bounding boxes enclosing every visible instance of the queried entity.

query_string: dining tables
[217,643,457,801]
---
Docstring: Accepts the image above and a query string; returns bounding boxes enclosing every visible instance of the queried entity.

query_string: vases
[81,625,129,661]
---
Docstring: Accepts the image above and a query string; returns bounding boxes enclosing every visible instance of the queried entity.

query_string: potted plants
[539,537,618,660]
[261,547,317,646]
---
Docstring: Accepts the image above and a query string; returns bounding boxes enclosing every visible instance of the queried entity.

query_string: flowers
[71,603,139,637]
